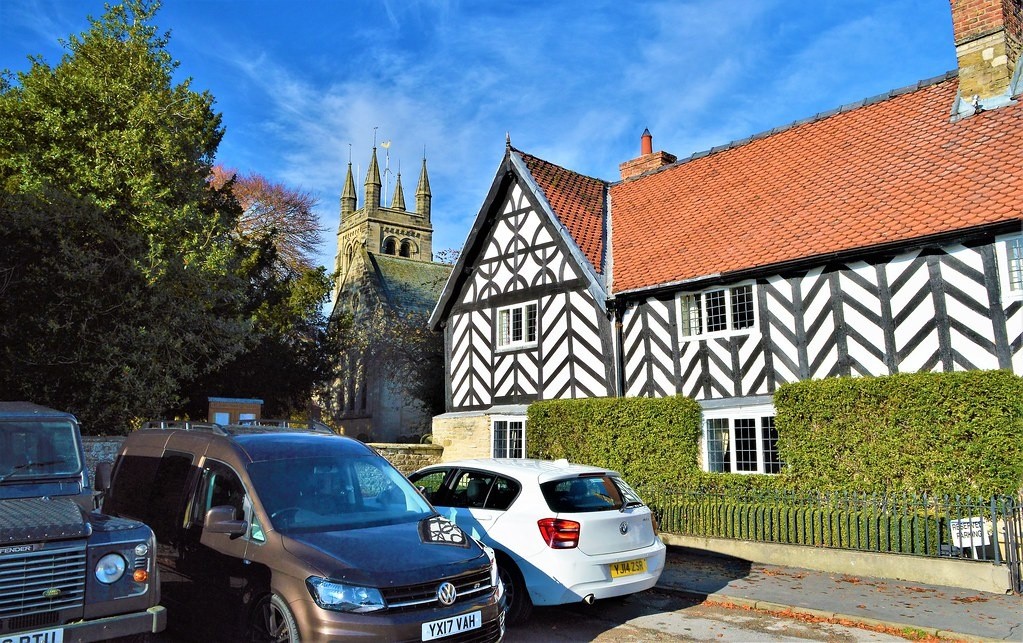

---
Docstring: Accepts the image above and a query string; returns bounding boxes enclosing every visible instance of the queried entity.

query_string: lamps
[971,94,981,113]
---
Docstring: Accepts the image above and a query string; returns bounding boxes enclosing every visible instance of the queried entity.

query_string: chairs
[569,482,589,497]
[467,479,488,507]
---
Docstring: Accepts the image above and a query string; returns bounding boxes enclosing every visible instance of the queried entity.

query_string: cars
[361,455,668,633]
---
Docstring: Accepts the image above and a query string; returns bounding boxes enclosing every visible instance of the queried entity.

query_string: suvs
[1,398,168,643]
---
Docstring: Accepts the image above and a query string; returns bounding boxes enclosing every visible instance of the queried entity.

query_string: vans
[99,419,510,643]
